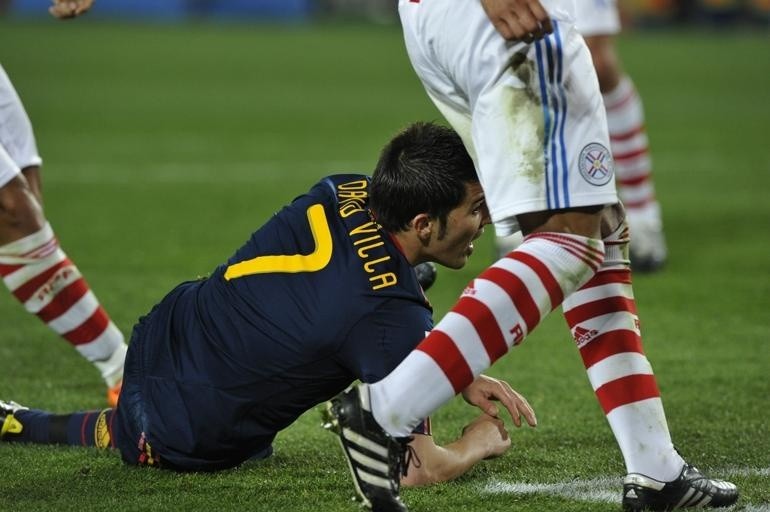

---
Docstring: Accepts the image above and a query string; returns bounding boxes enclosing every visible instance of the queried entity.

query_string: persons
[319,0,739,512]
[0,0,129,407]
[552,0,668,272]
[0,119,538,488]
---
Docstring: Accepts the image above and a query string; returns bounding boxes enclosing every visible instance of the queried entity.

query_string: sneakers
[317,382,414,512]
[622,460,738,512]
